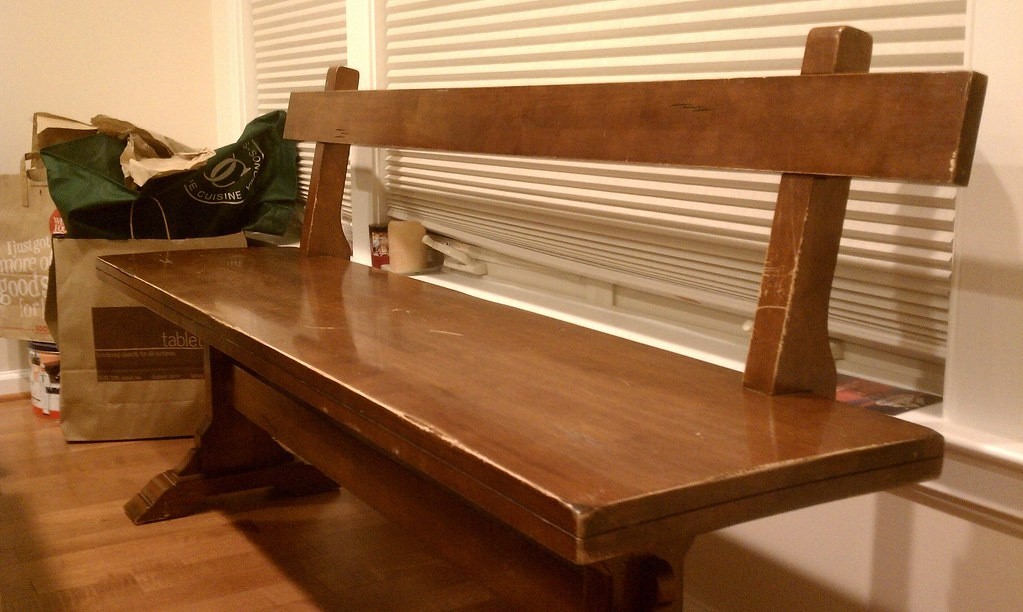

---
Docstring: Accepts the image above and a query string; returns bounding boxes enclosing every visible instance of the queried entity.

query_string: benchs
[96,26,988,611]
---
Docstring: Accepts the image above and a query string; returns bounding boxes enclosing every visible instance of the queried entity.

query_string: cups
[369,224,389,269]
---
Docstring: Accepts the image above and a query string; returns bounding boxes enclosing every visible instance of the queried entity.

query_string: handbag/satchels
[40,110,305,241]
[44,195,258,442]
[0,153,67,344]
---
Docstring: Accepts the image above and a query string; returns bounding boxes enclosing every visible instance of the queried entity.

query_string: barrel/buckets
[28,340,63,421]
